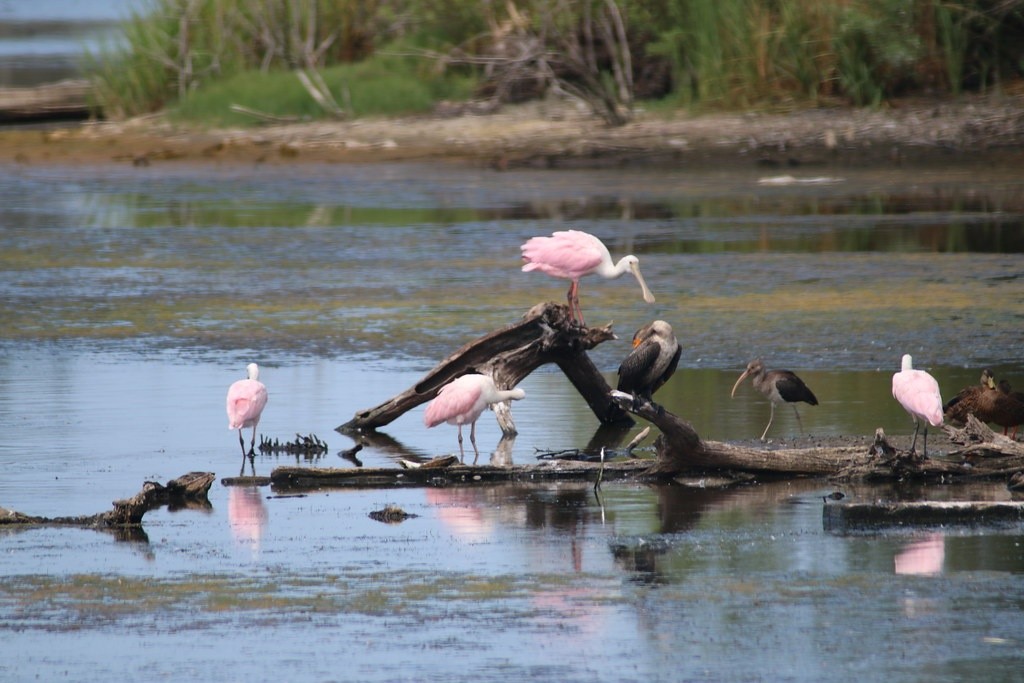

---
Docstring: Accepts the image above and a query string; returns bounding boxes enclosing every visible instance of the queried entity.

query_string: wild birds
[942,367,1024,441]
[616,319,683,410]
[225,363,268,459]
[518,226,658,327]
[423,373,526,456]
[890,353,946,457]
[730,359,820,441]
